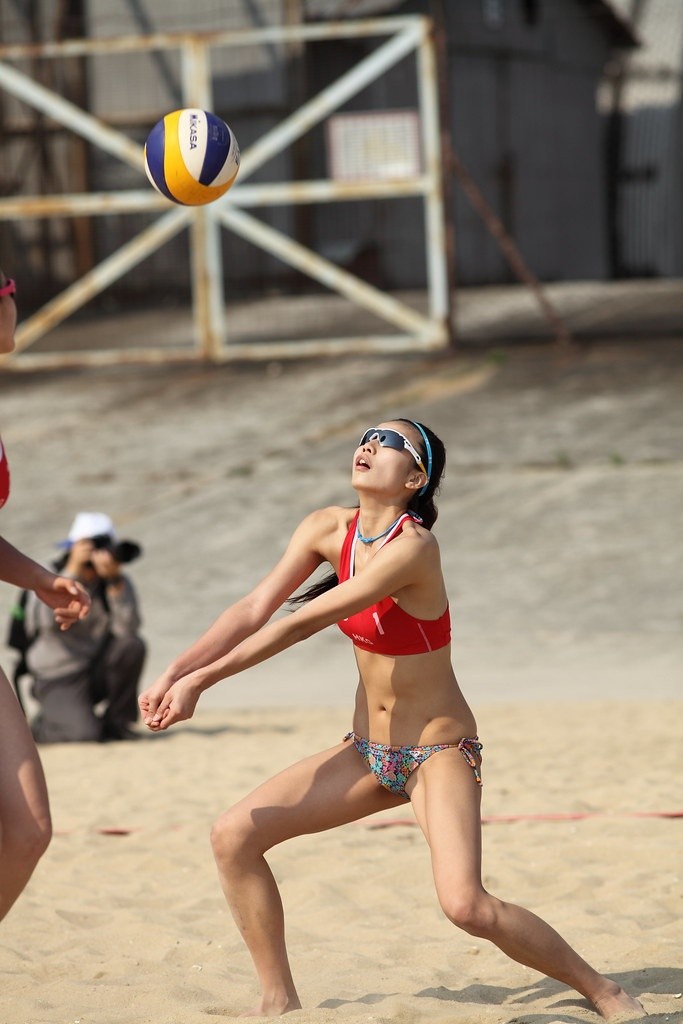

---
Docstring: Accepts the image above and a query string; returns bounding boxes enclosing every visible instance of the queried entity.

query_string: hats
[55,511,117,549]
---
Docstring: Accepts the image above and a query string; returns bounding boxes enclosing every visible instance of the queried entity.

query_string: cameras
[81,532,140,570]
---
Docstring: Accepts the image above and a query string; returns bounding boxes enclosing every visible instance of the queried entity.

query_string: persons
[10,510,146,744]
[140,419,651,1022]
[0,261,94,923]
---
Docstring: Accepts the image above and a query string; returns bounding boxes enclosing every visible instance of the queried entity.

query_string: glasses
[0,279,16,298]
[359,428,427,476]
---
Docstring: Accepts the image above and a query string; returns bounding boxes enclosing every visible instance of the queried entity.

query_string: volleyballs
[142,105,240,207]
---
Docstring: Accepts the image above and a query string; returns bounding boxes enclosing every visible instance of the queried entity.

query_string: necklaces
[358,518,399,544]
